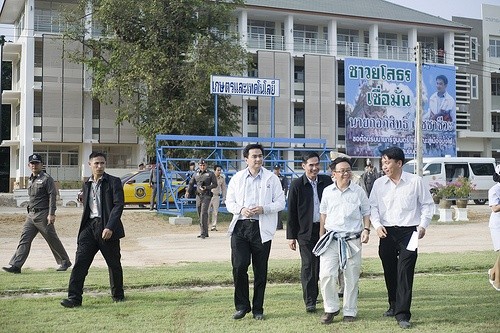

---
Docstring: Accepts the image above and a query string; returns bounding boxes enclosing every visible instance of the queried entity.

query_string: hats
[199,157,206,164]
[273,164,280,169]
[29,154,42,163]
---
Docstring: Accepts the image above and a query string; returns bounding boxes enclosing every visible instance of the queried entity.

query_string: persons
[428,75,456,123]
[185,157,218,240]
[186,160,197,204]
[208,165,227,231]
[361,164,377,197]
[224,144,286,322]
[312,157,371,323]
[273,164,288,230]
[286,152,334,314]
[329,151,368,298]
[368,147,435,329]
[138,162,147,207]
[2,154,72,272]
[60,150,126,308]
[149,161,165,210]
[487,165,500,291]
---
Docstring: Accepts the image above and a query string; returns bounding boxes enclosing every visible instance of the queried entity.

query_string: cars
[121,170,196,207]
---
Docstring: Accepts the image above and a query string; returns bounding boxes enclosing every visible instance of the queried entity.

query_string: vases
[433,196,467,208]
[13,188,30,207]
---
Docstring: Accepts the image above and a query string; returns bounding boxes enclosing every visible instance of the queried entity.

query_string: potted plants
[58,179,82,207]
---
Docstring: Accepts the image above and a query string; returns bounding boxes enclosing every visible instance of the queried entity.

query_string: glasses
[334,169,351,174]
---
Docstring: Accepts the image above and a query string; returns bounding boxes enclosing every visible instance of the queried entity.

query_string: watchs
[365,228,370,235]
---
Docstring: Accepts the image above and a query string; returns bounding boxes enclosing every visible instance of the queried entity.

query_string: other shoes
[198,234,208,239]
[306,305,315,311]
[57,262,72,271]
[3,266,21,274]
[323,309,340,322]
[60,299,82,307]
[397,319,410,328]
[344,316,355,321]
[488,269,495,284]
[234,306,251,319]
[253,313,263,320]
[492,282,500,292]
[383,309,395,316]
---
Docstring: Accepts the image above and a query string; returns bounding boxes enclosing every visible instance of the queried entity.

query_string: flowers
[430,175,475,198]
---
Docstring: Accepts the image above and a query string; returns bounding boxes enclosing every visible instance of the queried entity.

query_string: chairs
[451,167,465,181]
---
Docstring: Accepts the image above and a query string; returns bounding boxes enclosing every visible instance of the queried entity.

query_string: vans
[401,157,496,205]
